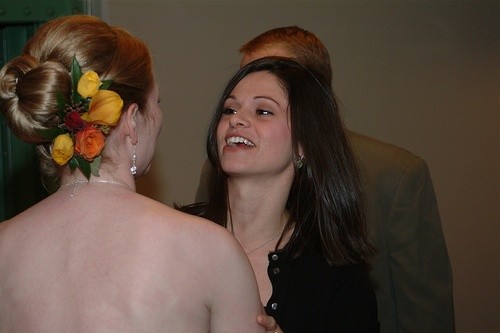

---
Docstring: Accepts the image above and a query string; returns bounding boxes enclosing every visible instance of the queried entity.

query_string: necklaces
[245,224,296,255]
[58,179,130,200]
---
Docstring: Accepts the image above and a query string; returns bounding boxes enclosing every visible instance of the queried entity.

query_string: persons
[0,13,266,333]
[238,24,458,333]
[174,55,383,333]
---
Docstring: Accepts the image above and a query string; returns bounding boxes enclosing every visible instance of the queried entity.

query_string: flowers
[33,52,124,184]
